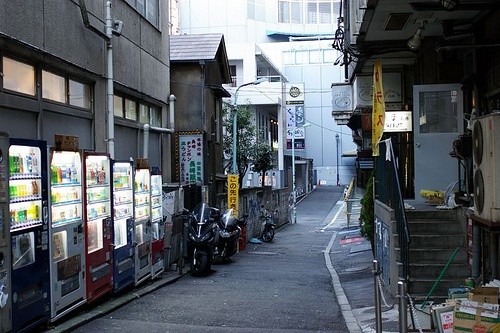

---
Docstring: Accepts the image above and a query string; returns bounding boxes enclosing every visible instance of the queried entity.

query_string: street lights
[233,77,269,175]
[335,133,339,186]
[289,123,311,224]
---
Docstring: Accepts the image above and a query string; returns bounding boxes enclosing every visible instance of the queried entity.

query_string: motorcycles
[183,202,223,277]
[213,207,249,264]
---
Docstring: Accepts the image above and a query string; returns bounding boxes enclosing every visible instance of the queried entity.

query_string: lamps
[128,157,134,163]
[407,16,436,50]
[442,0,459,8]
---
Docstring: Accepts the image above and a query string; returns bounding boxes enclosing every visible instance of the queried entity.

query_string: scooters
[259,205,278,242]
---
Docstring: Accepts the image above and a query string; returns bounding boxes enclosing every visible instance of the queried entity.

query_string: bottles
[87,189,109,202]
[50,162,78,184]
[27,153,38,173]
[51,189,78,203]
[9,204,40,222]
[86,161,106,186]
[9,151,23,174]
[9,180,38,197]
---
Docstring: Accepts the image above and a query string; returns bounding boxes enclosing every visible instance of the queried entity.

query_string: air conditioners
[471,114,500,221]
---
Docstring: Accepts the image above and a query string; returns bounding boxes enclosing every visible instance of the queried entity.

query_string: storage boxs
[431,285,500,333]
[420,188,445,205]
